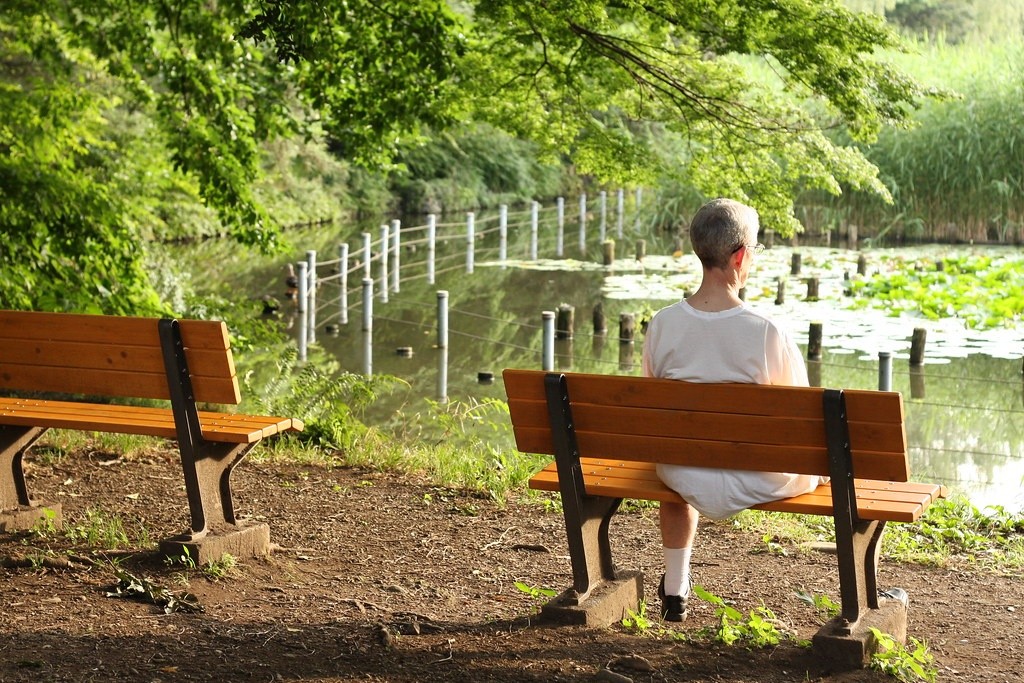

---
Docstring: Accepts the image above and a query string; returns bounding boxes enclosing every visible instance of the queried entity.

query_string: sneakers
[879,586,909,608]
[658,572,694,622]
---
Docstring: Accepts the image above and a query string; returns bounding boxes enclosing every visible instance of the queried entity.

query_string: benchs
[501,369,946,666]
[0,308,304,561]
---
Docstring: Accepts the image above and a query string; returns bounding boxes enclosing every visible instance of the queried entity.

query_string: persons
[642,197,828,622]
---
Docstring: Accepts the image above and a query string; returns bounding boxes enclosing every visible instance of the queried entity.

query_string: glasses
[731,243,765,257]
